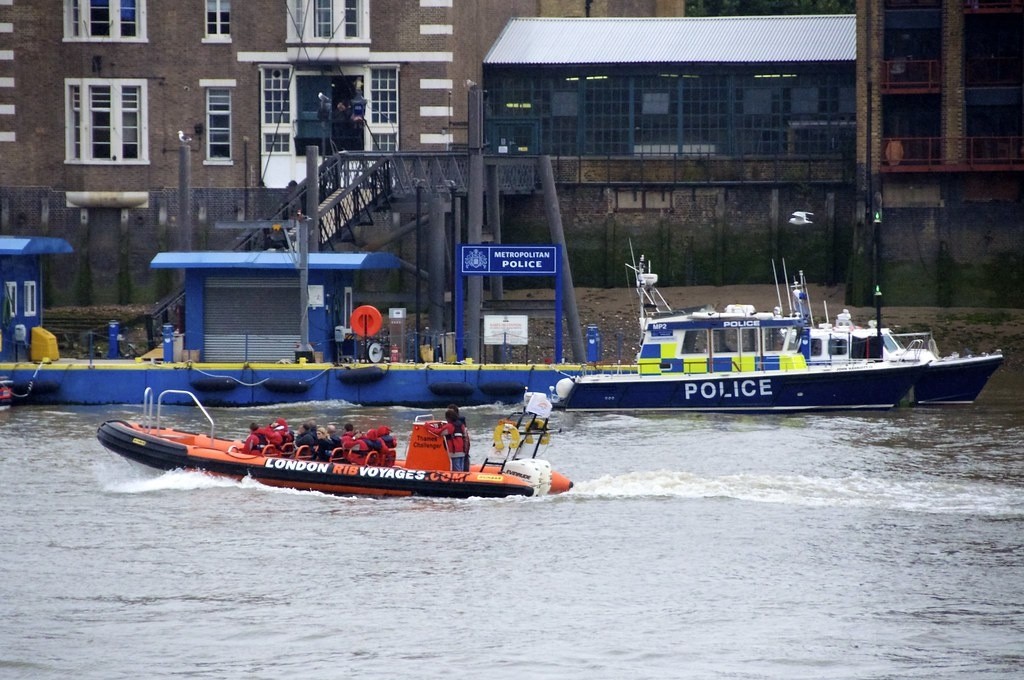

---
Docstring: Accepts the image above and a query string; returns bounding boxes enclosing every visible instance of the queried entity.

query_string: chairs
[281,444,294,458]
[330,447,344,463]
[262,444,275,457]
[382,450,396,467]
[364,451,379,466]
[296,446,310,459]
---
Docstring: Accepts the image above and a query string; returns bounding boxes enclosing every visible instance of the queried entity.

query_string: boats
[555,212,1004,414]
[0,229,638,407]
[97,386,573,499]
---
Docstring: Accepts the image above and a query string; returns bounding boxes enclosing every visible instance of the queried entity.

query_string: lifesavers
[524,420,551,445]
[494,424,521,450]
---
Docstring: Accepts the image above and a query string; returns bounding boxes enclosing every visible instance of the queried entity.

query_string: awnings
[483,17,857,64]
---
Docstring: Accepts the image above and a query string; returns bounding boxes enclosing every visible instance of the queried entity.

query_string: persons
[331,100,363,151]
[425,404,470,472]
[238,417,397,467]
